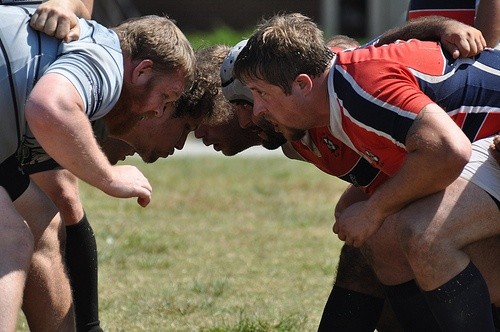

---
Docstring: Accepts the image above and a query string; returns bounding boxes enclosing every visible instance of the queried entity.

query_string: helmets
[220,39,254,104]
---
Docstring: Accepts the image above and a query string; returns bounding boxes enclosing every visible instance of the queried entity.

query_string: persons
[0,3,196,332]
[232,11,500,332]
[0,0,500,332]
